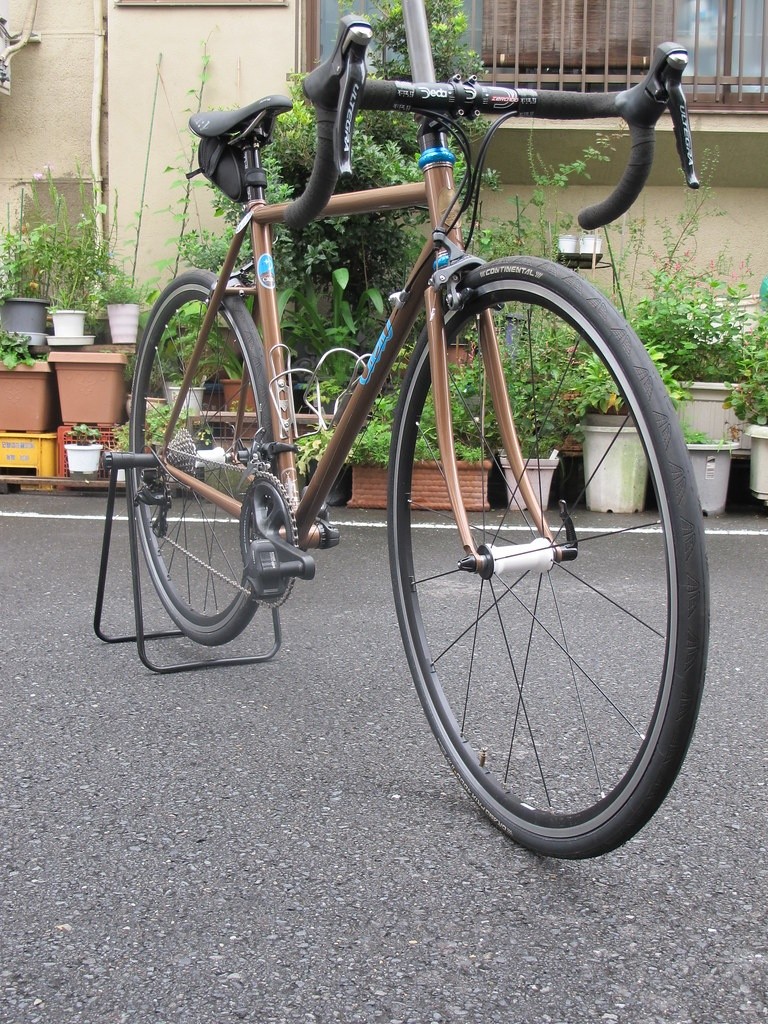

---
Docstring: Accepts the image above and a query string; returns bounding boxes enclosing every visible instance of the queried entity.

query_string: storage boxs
[55,425,121,492]
[0,432,61,492]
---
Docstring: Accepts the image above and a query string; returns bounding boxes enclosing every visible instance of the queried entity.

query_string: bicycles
[87,16,712,858]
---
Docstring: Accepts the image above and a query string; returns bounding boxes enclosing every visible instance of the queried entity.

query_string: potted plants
[262,0,768,519]
[111,407,193,480]
[0,41,261,438]
